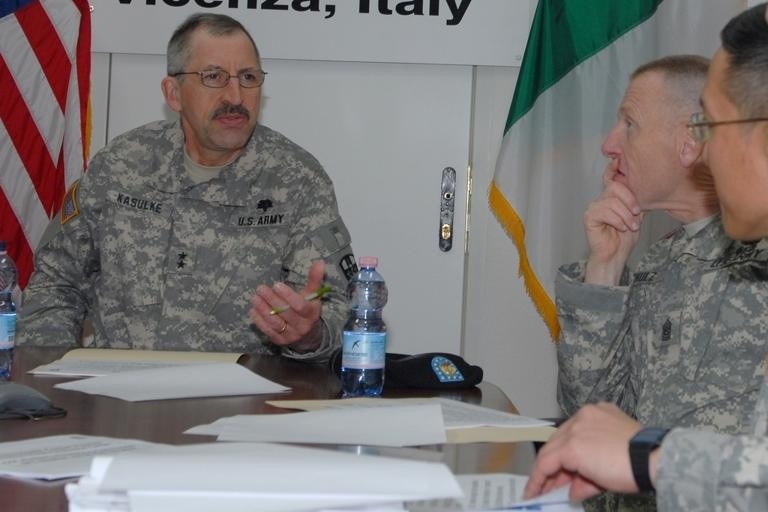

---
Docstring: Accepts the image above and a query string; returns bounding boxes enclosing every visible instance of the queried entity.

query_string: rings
[275,319,288,334]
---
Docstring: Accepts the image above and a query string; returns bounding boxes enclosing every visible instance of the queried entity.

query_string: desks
[2,342,538,511]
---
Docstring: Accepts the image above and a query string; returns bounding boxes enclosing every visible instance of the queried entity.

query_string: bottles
[0,241,19,381]
[340,254,390,396]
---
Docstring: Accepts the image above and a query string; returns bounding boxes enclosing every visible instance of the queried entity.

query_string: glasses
[176,69,268,89]
[686,110,766,139]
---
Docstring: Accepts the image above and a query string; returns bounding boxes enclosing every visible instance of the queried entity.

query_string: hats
[330,347,483,391]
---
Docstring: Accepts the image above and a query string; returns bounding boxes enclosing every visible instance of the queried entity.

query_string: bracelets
[628,428,672,492]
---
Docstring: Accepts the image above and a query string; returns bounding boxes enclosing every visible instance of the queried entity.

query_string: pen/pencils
[270,285,333,315]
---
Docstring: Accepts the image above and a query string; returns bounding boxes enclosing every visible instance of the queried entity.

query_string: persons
[550,54,726,512]
[13,12,357,366]
[520,2,768,511]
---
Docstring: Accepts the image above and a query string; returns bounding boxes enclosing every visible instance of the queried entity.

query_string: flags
[0,1,93,293]
[487,2,766,349]
